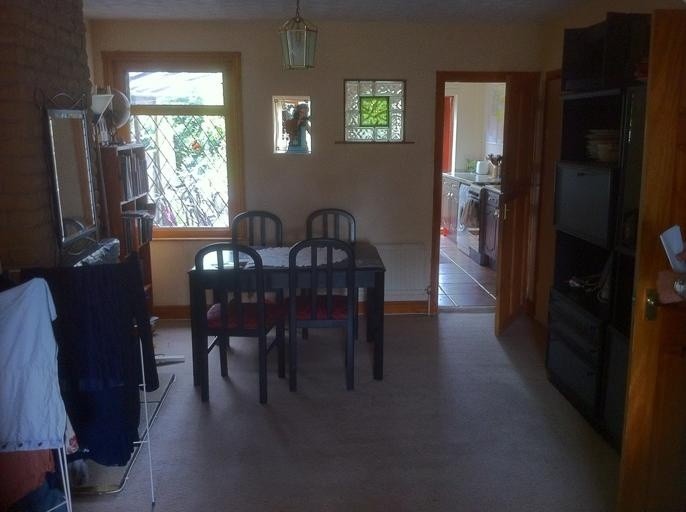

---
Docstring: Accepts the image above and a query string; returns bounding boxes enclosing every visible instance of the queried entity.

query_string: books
[120,152,157,253]
[659,224,686,274]
[97,117,109,148]
[584,126,620,162]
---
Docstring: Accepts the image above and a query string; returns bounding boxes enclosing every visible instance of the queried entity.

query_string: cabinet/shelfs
[545,84,647,463]
[101,140,160,337]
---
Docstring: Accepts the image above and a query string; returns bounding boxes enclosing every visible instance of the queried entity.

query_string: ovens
[469,190,485,265]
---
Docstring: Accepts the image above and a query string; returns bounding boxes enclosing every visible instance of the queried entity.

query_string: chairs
[195,208,357,404]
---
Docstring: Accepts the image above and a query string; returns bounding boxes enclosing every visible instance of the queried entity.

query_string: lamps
[278,0,319,70]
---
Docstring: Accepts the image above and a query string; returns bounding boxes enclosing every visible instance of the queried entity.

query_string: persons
[653,251,686,305]
[283,103,311,154]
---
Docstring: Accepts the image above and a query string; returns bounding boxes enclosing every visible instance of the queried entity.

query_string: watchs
[672,277,686,297]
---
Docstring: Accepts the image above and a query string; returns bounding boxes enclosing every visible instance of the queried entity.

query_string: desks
[187,240,385,387]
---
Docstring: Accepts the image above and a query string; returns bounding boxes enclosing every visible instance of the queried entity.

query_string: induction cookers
[472,182,502,187]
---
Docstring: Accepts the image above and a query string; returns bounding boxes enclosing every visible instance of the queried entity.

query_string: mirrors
[271,95,311,154]
[35,86,105,263]
